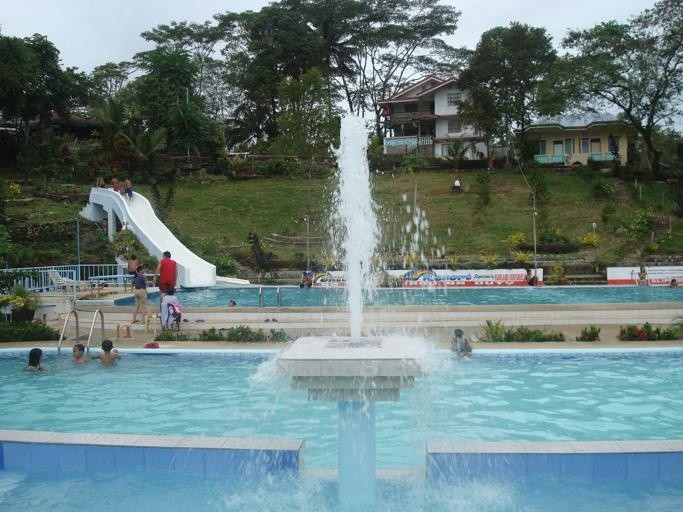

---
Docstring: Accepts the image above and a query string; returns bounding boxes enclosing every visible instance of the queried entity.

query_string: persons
[128,254,140,273]
[90,340,122,368]
[521,269,541,288]
[128,263,150,324]
[68,344,88,366]
[449,329,472,363]
[149,250,178,315]
[21,348,46,373]
[634,264,651,286]
[299,270,313,288]
[94,174,132,204]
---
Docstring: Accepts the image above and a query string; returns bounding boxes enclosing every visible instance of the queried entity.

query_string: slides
[87,185,218,291]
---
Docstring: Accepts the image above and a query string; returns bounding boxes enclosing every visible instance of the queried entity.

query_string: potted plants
[0,284,41,322]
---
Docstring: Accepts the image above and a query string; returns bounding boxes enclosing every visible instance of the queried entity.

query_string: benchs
[88,274,160,294]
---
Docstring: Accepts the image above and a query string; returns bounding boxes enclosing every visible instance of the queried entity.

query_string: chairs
[47,271,74,292]
